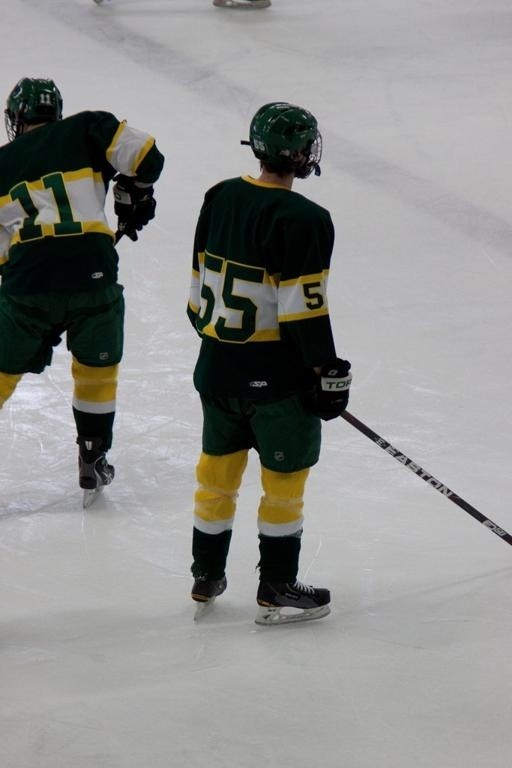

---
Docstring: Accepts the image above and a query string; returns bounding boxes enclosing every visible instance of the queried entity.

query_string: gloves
[111,175,156,240]
[311,355,351,420]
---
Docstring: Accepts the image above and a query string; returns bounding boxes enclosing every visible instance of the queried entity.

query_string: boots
[192,559,228,604]
[77,439,115,492]
[256,564,331,611]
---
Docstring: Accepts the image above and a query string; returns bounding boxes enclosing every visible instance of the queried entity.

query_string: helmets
[5,77,61,141]
[251,101,321,180]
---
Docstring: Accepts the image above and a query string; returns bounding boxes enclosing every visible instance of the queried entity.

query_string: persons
[185,103,352,609]
[0,77,165,490]
